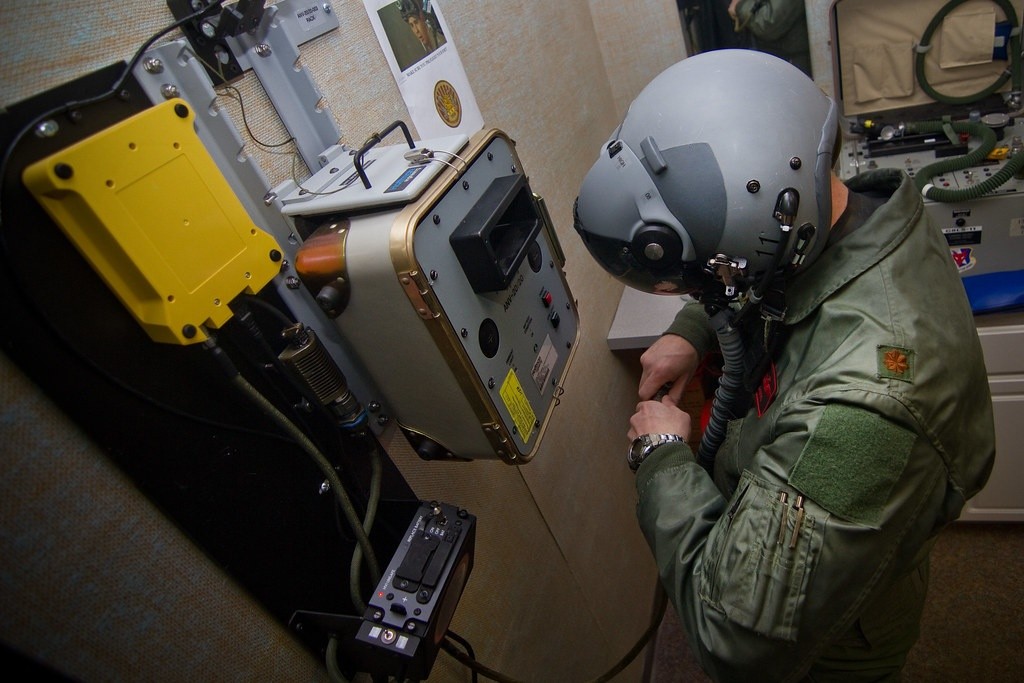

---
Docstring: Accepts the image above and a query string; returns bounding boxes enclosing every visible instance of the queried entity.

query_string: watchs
[627,432,687,475]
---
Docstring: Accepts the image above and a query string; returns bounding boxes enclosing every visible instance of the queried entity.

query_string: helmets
[396,0,427,20]
[573,49,840,291]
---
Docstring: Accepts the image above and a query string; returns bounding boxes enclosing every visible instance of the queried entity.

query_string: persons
[573,49,996,683]
[729,0,813,79]
[396,0,446,54]
